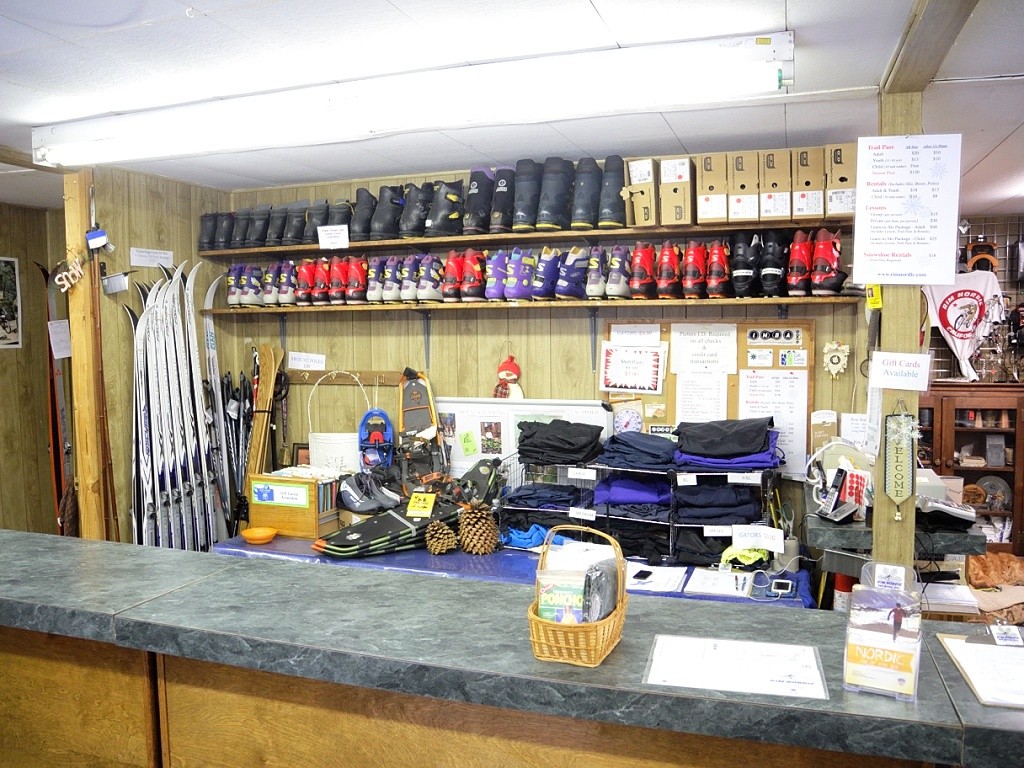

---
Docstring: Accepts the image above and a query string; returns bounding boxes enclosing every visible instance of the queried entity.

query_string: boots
[198,155,867,306]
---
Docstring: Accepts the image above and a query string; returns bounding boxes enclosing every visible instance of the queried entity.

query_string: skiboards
[33,258,67,539]
[236,342,285,534]
[118,259,234,554]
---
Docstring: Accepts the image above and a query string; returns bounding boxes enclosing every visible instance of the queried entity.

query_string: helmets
[719,543,771,568]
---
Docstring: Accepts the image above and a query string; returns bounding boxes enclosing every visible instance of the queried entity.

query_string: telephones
[815,468,860,525]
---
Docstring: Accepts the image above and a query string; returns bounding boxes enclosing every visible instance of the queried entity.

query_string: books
[845,583,922,694]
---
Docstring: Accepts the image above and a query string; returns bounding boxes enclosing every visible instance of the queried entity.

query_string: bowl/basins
[240,527,278,545]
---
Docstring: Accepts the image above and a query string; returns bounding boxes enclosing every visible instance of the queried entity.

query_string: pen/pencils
[742,576,747,591]
[735,575,738,590]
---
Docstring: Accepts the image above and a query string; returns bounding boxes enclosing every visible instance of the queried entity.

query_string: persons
[887,603,906,643]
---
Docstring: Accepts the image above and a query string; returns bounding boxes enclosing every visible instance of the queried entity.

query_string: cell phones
[633,570,652,580]
[771,579,792,594]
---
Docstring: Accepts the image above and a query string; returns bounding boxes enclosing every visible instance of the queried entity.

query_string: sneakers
[336,464,415,515]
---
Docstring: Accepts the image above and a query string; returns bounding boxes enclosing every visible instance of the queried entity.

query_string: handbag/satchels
[528,523,630,668]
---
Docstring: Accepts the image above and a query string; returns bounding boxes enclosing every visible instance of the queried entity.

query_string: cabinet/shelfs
[196,227,865,314]
[489,457,785,576]
[915,379,1024,557]
